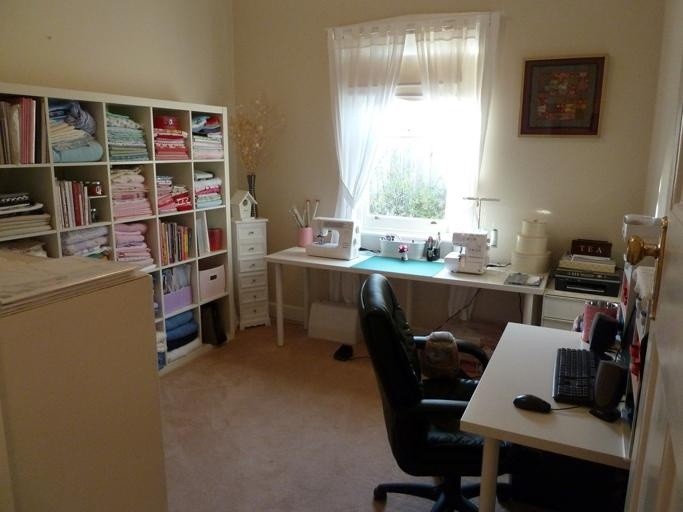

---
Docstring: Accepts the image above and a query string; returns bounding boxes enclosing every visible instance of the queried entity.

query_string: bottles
[0,192,44,216]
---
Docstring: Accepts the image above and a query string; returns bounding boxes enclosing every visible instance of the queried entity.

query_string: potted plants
[516,55,603,136]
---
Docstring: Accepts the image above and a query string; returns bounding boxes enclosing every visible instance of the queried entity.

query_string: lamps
[360,271,517,512]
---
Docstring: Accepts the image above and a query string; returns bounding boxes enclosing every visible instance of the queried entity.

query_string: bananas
[552,348,597,407]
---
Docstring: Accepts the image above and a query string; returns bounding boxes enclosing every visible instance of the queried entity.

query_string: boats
[589,312,619,353]
[589,360,628,423]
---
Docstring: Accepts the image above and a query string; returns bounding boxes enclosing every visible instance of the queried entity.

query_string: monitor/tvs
[55,178,91,230]
[0,97,47,164]
[504,273,544,287]
[160,220,192,267]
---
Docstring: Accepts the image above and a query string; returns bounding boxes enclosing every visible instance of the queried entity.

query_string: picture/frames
[458,323,633,512]
[261,245,550,347]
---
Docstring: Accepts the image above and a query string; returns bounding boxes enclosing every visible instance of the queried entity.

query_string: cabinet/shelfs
[298,227,312,247]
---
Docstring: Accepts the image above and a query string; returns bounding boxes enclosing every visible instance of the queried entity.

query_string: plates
[1,245,171,511]
[230,218,273,329]
[540,276,623,333]
[1,80,238,378]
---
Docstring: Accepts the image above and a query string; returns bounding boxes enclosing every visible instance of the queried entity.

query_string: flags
[246,174,259,217]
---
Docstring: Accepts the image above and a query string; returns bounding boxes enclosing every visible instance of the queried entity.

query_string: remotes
[513,394,551,413]
